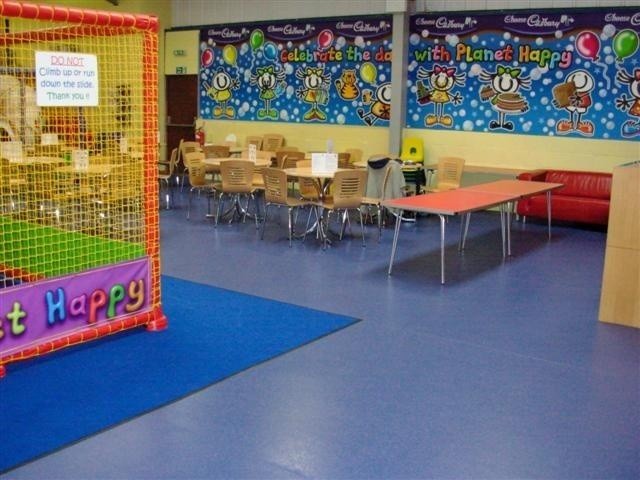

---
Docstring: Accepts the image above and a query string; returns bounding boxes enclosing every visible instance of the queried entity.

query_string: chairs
[214,158,260,229]
[397,137,426,220]
[344,167,392,235]
[322,169,368,246]
[0,135,182,222]
[243,155,301,225]
[186,154,222,219]
[261,168,319,247]
[423,158,464,221]
[297,160,324,198]
[182,134,360,165]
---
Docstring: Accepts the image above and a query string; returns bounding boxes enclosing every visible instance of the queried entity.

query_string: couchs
[515,169,612,231]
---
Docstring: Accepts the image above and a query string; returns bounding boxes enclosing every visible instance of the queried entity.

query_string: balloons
[201,49,214,67]
[575,30,600,60]
[263,41,277,62]
[318,30,334,50]
[250,29,264,50]
[360,62,377,85]
[222,44,237,65]
[613,30,640,62]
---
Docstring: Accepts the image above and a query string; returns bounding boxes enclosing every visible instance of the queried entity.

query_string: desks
[380,178,565,284]
[598,157,640,331]
[203,158,270,220]
[282,168,350,242]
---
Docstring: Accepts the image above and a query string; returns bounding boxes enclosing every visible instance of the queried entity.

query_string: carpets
[0,275,363,473]
